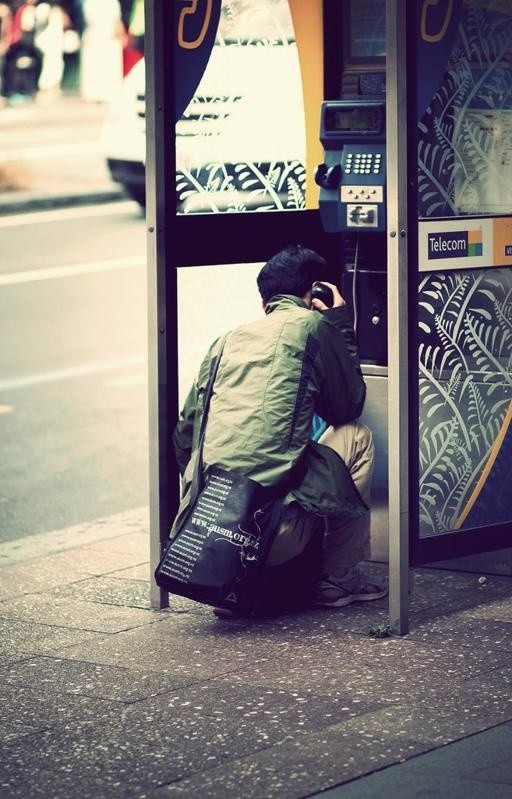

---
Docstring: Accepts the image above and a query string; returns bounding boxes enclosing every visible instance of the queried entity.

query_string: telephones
[312,283,333,309]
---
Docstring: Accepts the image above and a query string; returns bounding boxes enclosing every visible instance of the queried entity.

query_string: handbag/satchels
[155,467,282,614]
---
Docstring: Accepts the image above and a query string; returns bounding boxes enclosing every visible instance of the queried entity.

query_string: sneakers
[315,565,388,607]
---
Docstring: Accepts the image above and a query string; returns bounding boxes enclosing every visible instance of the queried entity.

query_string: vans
[103,0,305,213]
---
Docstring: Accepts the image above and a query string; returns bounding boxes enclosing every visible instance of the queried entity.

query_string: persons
[0,0,142,111]
[175,246,389,617]
